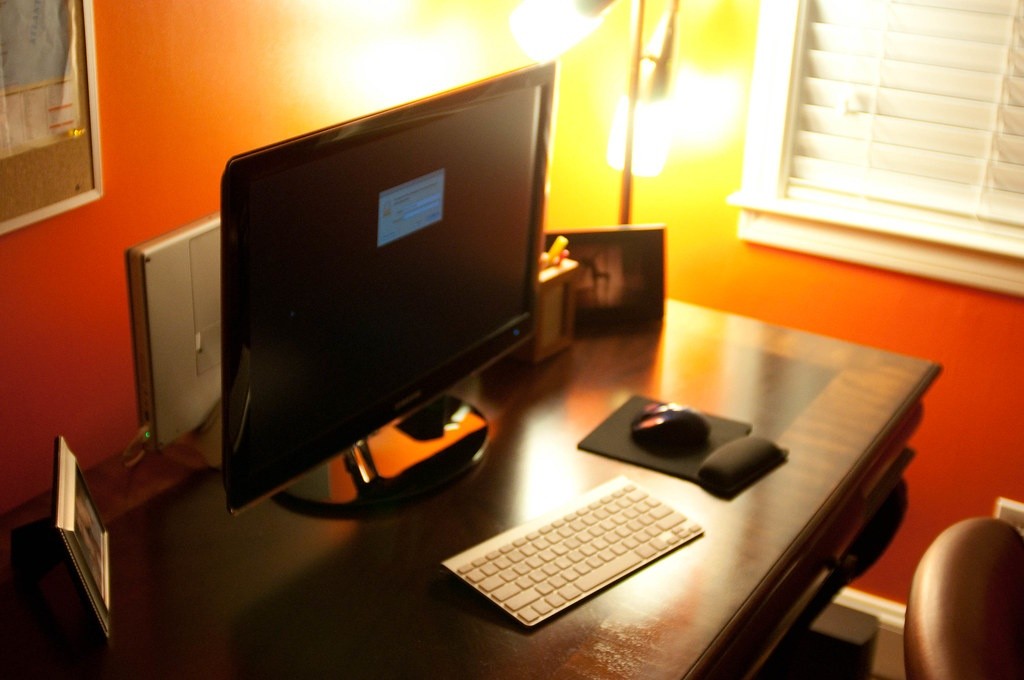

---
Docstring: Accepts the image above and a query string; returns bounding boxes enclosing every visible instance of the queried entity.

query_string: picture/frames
[544,225,666,328]
[51,435,114,640]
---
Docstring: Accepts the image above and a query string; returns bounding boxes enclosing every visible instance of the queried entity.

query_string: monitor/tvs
[221,59,556,518]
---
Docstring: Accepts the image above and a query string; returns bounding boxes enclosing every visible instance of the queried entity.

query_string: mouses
[631,402,715,450]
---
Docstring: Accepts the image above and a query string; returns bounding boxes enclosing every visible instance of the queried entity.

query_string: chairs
[904,516,1024,680]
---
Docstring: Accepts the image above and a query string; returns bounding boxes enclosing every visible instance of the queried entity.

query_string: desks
[0,300,942,680]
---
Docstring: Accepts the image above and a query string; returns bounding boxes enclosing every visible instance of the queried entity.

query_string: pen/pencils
[541,235,570,271]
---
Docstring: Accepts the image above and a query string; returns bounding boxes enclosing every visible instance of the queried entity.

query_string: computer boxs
[125,209,220,452]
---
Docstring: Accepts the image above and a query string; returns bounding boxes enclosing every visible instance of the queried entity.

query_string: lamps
[510,0,687,225]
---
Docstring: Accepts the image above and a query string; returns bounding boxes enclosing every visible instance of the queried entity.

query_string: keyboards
[442,474,704,627]
